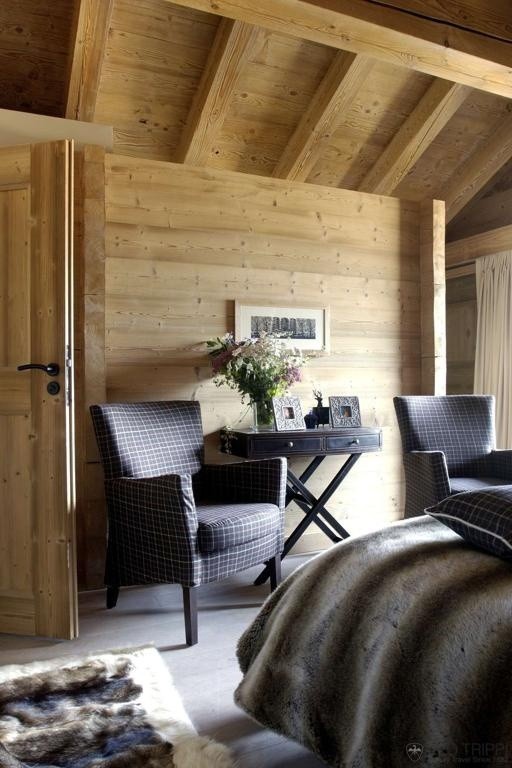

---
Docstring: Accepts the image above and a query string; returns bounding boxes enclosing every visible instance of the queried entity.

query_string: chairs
[393,395,512,519]
[89,401,288,645]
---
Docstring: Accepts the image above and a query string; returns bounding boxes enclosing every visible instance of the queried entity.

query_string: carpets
[0,645,239,768]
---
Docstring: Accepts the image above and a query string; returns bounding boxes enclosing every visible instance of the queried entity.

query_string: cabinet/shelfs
[220,423,382,585]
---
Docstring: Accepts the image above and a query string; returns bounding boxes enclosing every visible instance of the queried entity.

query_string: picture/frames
[271,397,306,432]
[234,297,329,357]
[329,396,362,429]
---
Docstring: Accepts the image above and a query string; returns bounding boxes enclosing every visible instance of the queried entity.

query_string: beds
[236,516,512,768]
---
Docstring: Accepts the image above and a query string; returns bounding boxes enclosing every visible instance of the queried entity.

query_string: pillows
[424,485,512,560]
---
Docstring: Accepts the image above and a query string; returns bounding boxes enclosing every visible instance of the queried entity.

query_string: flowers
[207,333,301,425]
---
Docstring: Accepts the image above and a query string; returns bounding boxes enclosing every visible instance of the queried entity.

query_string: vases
[253,399,275,432]
